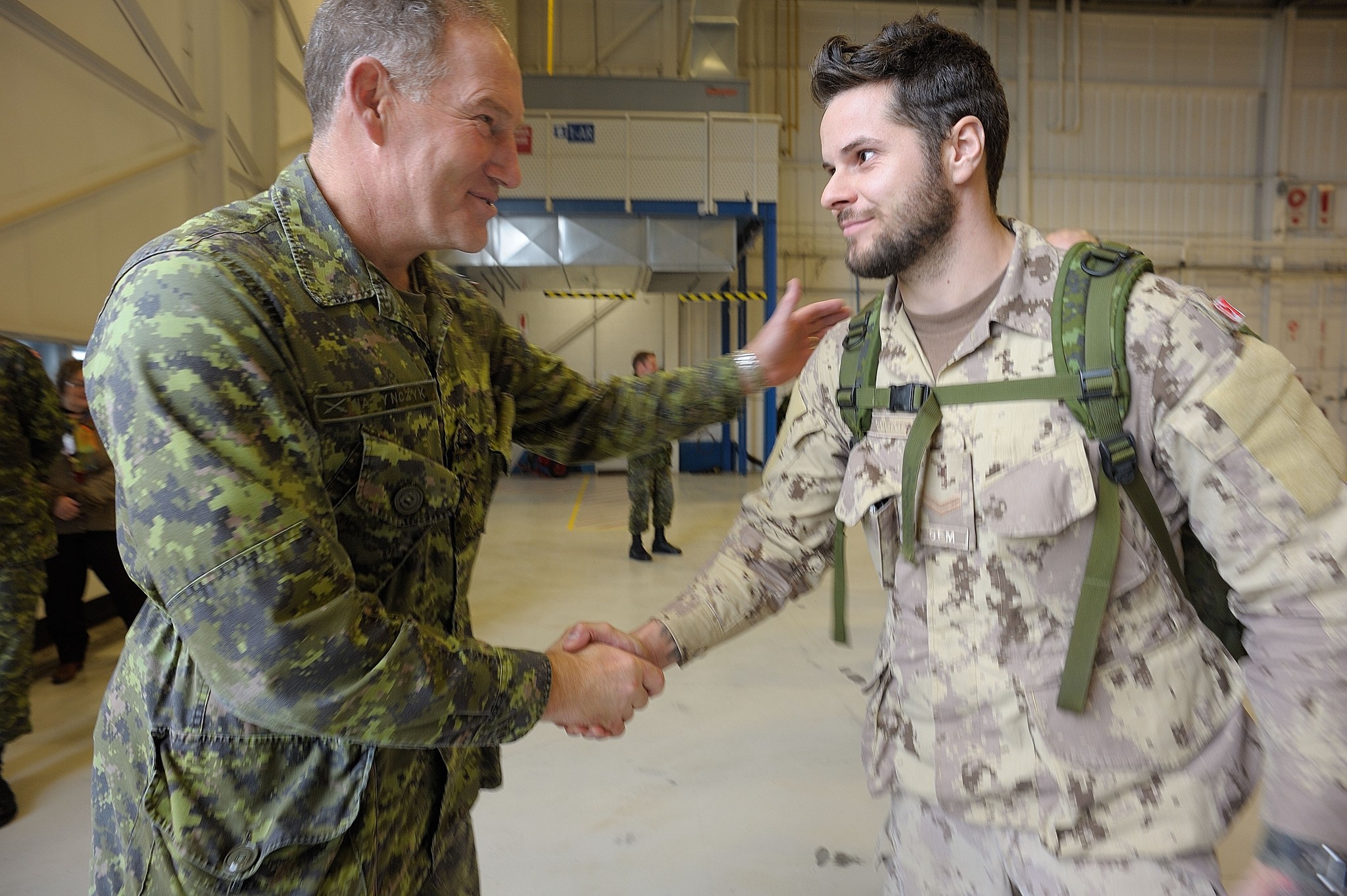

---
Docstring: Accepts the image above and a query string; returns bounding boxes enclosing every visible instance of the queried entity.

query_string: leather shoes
[630,540,652,562]
[652,540,681,554]
[53,661,82,683]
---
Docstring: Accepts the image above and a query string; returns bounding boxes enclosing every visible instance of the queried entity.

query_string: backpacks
[827,239,1256,718]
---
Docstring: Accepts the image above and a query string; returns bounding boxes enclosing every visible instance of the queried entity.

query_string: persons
[1,331,65,826]
[550,13,1347,895]
[628,351,682,561]
[78,4,859,895]
[50,358,146,685]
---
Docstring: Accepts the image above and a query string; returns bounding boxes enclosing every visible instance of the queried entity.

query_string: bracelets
[1255,828,1347,895]
[732,350,770,394]
